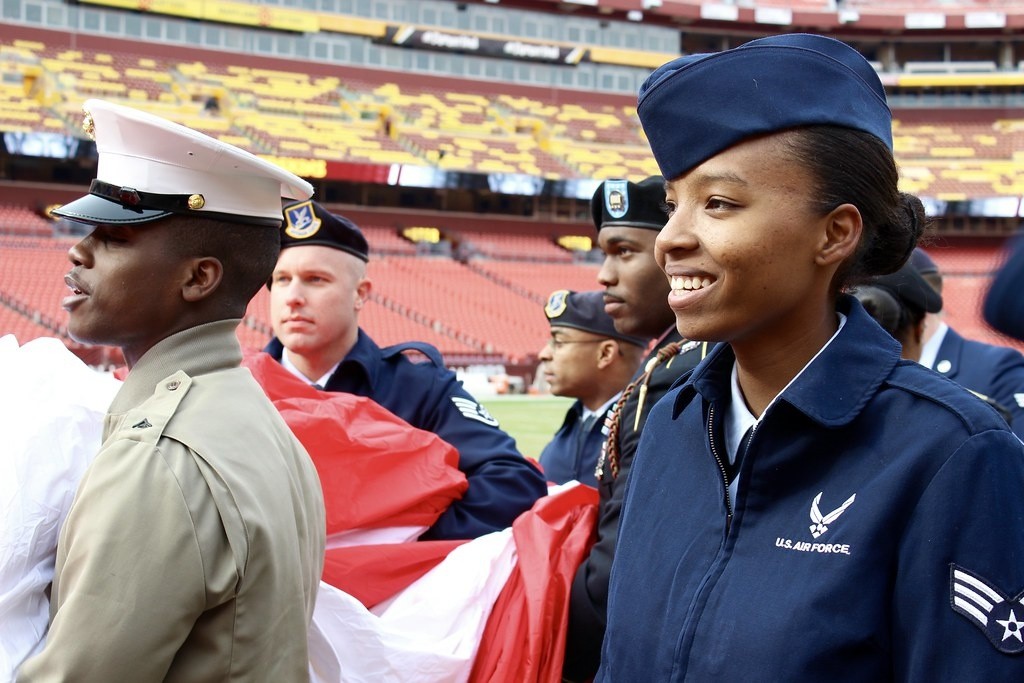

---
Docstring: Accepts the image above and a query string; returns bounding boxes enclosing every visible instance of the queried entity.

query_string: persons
[562,175,718,683]
[843,249,1024,442]
[260,202,547,541]
[536,289,652,485]
[592,34,1024,683]
[12,99,326,683]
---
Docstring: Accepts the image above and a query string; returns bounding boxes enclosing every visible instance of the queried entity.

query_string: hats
[903,248,938,273]
[589,175,670,232]
[544,289,651,349]
[266,199,370,291]
[637,33,893,180]
[50,100,314,226]
[877,268,942,314]
